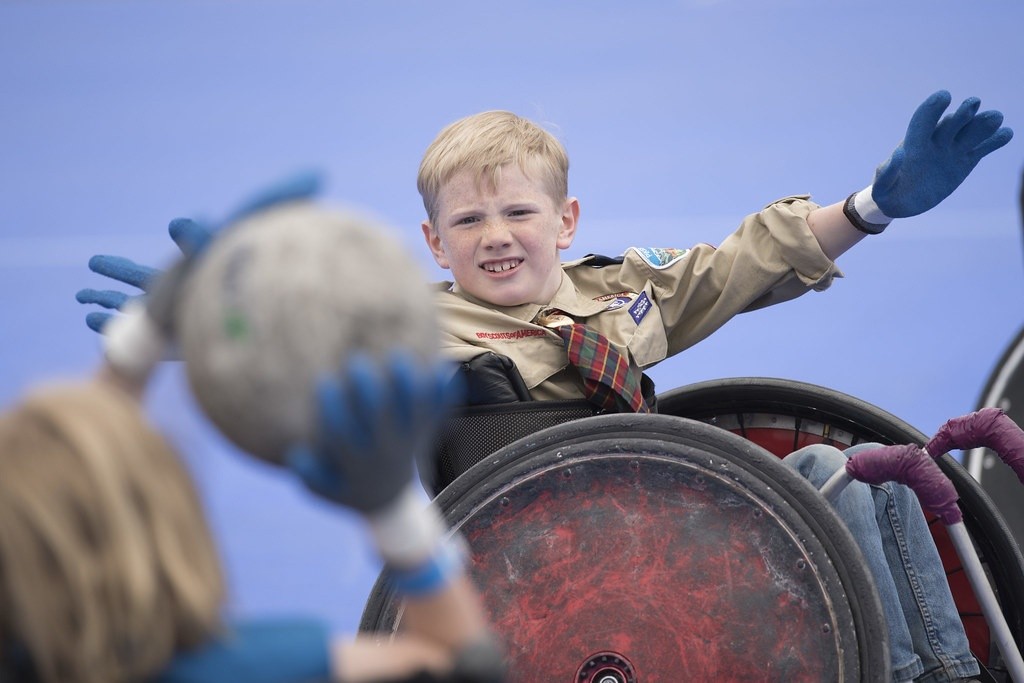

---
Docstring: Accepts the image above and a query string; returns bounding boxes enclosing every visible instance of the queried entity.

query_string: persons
[73,89,1015,683]
[0,174,512,683]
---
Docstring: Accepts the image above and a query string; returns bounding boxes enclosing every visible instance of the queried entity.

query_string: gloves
[75,216,212,361]
[842,90,1013,235]
[288,354,466,596]
[95,171,316,381]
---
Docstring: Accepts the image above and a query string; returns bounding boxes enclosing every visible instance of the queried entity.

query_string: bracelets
[390,533,471,597]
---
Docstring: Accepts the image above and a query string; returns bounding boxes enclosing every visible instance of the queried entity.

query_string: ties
[530,311,649,413]
